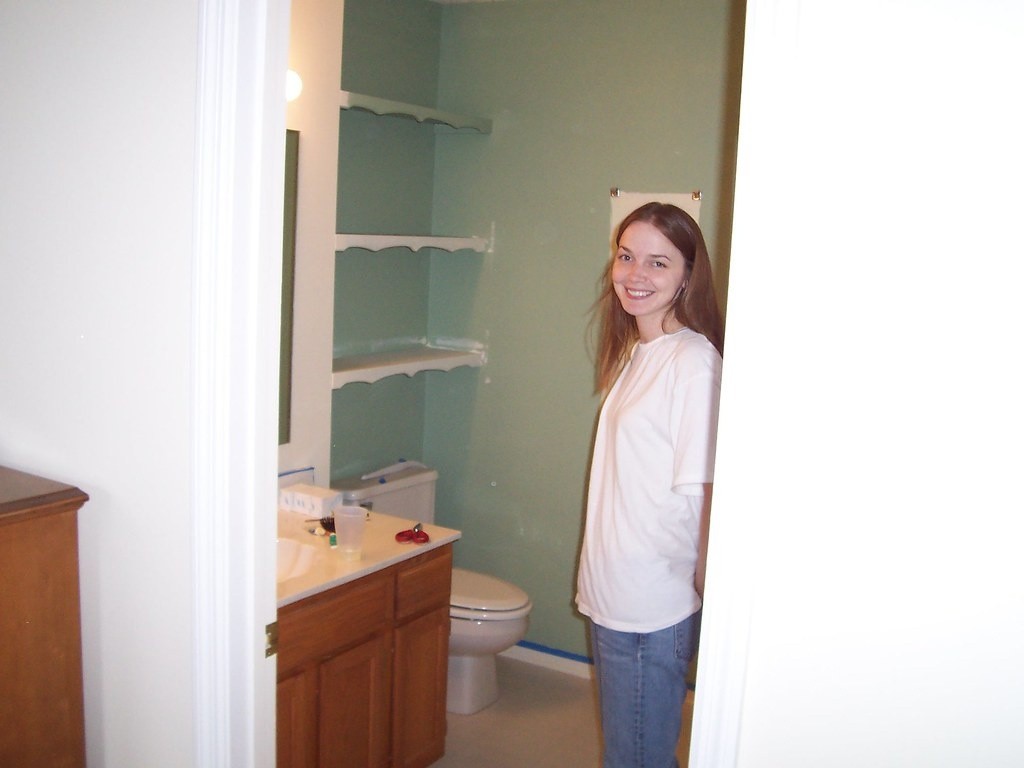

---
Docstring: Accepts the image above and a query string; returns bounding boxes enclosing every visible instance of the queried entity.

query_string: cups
[333,506,367,552]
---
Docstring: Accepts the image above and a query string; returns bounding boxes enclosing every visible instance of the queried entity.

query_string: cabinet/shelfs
[277,543,454,768]
[330,87,494,392]
[0,465,91,768]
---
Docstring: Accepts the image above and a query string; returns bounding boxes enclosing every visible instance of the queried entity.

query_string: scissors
[395,523,430,545]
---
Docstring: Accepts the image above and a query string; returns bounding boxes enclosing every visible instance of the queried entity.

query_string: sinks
[278,538,313,585]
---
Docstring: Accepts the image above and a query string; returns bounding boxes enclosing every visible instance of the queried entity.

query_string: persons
[574,201,723,768]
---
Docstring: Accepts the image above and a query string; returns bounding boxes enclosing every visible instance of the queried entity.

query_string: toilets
[330,459,532,713]
